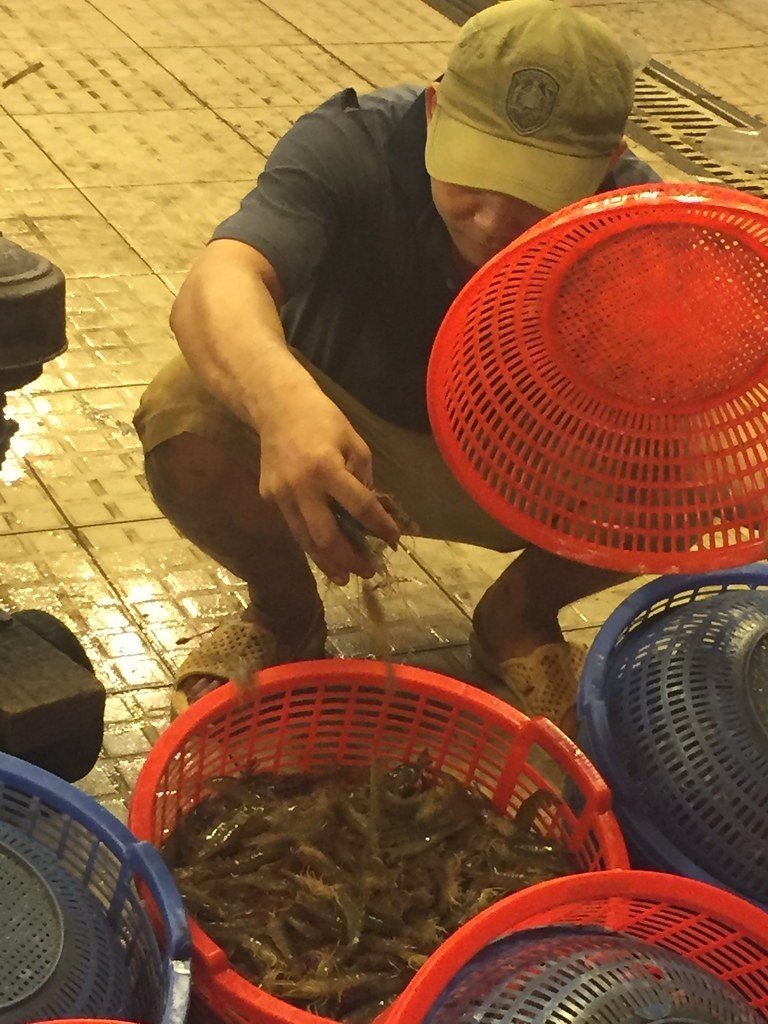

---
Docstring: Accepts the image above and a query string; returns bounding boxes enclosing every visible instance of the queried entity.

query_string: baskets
[2,657,766,1022]
[575,578,768,907]
[424,184,768,574]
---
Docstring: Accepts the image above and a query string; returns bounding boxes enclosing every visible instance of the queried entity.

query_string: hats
[422,0,637,215]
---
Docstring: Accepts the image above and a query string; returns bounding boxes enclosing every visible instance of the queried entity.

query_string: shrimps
[157,749,582,1023]
[333,493,422,591]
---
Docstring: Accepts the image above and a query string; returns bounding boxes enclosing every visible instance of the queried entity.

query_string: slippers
[169,618,328,728]
[462,631,592,738]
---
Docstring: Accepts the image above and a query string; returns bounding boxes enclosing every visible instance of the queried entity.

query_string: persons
[133,1,766,755]
